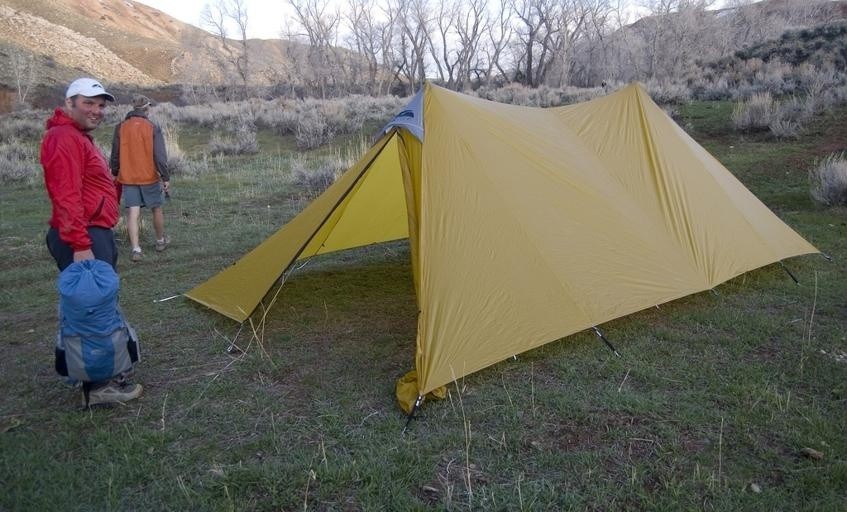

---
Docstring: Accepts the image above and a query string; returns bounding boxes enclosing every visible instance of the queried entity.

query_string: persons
[40,77,145,407]
[109,93,170,262]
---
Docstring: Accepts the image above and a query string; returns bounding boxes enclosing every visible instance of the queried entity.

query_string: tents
[183,79,823,415]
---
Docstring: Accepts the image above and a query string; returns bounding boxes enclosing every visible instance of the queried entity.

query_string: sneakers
[129,248,143,261]
[156,235,170,251]
[82,380,143,407]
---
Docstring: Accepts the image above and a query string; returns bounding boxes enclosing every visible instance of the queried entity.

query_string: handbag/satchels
[55,258,142,387]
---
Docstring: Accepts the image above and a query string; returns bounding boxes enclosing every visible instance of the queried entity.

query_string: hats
[66,78,116,102]
